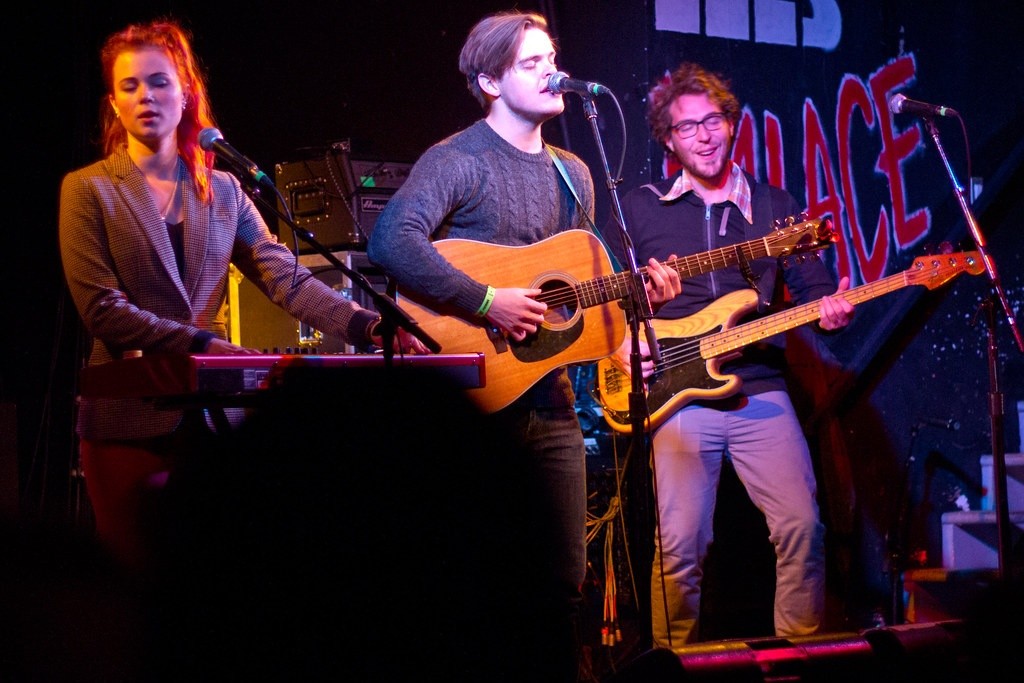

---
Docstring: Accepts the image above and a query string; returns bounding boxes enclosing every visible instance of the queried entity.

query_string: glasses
[669,111,727,138]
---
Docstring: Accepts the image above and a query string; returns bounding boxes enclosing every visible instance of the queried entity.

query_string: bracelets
[368,316,382,348]
[472,285,496,316]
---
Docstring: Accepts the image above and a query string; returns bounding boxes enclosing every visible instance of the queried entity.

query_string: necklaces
[161,164,181,219]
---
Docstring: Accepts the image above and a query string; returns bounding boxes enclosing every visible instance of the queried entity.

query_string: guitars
[597,240,994,436]
[394,212,840,416]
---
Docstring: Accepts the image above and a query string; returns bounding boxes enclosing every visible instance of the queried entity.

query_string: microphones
[888,94,959,117]
[548,71,610,97]
[918,415,961,430]
[198,126,274,186]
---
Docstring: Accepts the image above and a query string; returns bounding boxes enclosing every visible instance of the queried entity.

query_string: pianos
[77,345,487,401]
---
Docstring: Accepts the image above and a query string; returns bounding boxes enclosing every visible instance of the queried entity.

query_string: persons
[59,22,432,623]
[601,62,853,649]
[367,15,681,663]
